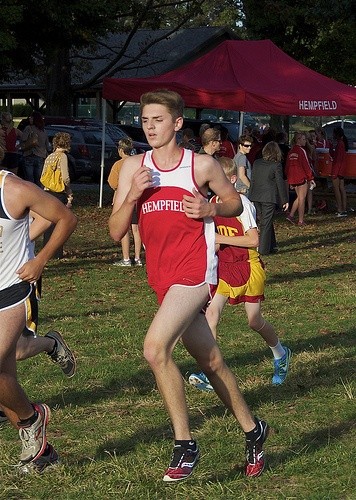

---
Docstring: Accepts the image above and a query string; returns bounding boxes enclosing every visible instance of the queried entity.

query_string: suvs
[14,110,270,185]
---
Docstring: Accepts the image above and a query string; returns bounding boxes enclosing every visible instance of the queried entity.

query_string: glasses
[244,145,252,147]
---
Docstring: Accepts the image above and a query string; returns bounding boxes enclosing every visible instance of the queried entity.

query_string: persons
[286,133,316,226]
[107,88,269,481]
[232,134,253,199]
[189,157,291,394]
[329,127,348,217]
[179,123,336,215]
[108,139,144,266]
[41,132,73,258]
[198,128,222,201]
[0,128,79,465]
[0,111,50,188]
[248,141,289,255]
[0,210,75,421]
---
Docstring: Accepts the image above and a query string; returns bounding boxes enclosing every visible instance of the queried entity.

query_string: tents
[99,40,356,208]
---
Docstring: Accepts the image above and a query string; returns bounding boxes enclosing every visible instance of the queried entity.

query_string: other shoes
[286,216,295,224]
[335,210,347,217]
[297,222,306,226]
[133,259,143,267]
[113,259,131,267]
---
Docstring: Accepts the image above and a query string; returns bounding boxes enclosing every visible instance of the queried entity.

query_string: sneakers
[272,345,291,385]
[25,444,58,474]
[162,445,200,482]
[18,401,50,462]
[189,373,214,392]
[44,330,77,379]
[245,418,270,476]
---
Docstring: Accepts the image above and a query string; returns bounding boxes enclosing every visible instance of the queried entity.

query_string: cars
[311,119,356,182]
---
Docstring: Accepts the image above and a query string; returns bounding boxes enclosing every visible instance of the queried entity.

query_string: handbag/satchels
[40,153,65,192]
[22,127,37,156]
